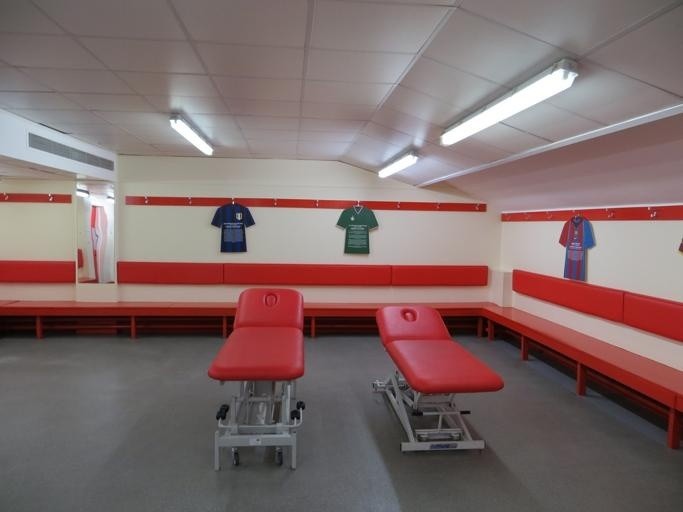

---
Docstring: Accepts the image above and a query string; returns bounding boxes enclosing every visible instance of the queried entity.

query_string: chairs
[371,301,505,453]
[209,287,304,471]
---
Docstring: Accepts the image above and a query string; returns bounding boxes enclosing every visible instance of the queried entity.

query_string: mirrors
[77,177,116,288]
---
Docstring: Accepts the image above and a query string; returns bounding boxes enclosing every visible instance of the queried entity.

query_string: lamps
[372,146,419,186]
[434,55,582,149]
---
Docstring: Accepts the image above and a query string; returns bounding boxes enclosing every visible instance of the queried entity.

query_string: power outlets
[167,111,217,163]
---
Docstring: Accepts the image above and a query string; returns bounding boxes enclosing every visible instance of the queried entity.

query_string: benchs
[0,259,495,338]
[480,302,683,453]
